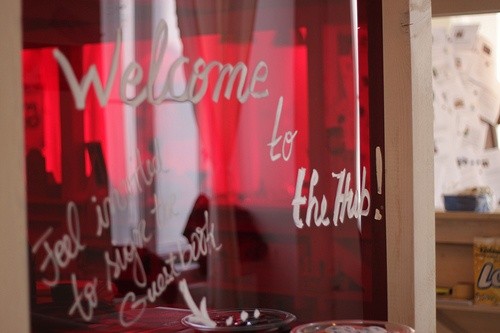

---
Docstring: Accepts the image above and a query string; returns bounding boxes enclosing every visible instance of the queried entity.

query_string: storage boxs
[442,193,496,213]
